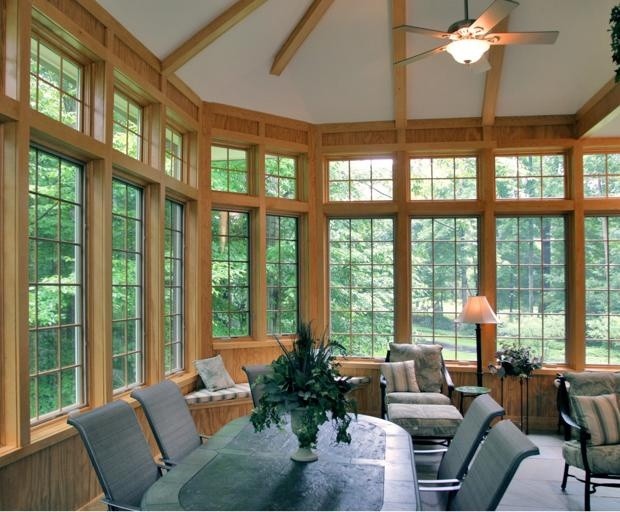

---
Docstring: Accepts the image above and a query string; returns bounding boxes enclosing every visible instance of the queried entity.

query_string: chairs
[553,369,620,511]
[130,379,212,471]
[380,342,465,454]
[241,364,283,410]
[415,419,540,512]
[66,396,176,511]
[409,393,506,512]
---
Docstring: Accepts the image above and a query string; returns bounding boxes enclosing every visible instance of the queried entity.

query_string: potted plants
[246,317,362,468]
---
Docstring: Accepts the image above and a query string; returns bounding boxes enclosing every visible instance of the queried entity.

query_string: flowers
[486,340,543,384]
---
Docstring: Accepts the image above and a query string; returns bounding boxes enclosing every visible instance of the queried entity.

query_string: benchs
[183,382,256,439]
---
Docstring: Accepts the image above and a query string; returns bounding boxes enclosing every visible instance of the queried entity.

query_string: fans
[388,0,560,76]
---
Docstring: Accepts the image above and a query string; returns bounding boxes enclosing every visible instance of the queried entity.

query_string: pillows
[192,353,236,393]
[569,393,619,448]
[378,359,423,394]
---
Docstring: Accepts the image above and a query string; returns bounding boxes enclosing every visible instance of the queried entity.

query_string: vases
[501,361,532,379]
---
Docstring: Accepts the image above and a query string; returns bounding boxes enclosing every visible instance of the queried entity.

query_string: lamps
[444,39,492,65]
[454,295,505,386]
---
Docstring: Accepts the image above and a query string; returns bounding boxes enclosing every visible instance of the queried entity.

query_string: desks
[138,405,420,511]
[454,385,492,414]
